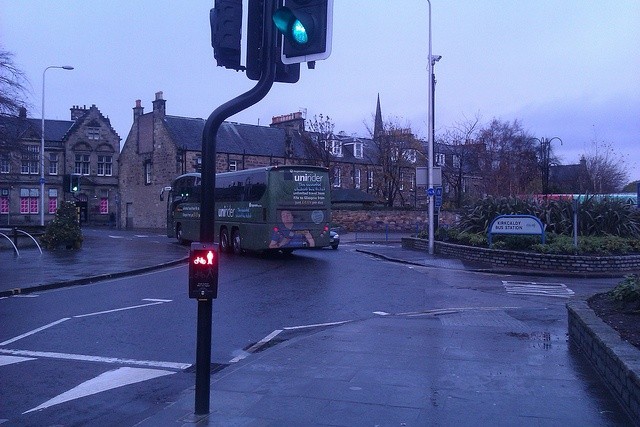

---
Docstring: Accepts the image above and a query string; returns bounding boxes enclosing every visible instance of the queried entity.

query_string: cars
[330,230,339,248]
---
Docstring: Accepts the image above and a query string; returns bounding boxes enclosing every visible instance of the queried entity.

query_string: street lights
[40,65,74,225]
[531,136,563,195]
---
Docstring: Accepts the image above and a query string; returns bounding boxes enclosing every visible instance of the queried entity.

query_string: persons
[268,209,315,249]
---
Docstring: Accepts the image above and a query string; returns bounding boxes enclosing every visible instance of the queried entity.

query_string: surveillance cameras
[427,54,442,61]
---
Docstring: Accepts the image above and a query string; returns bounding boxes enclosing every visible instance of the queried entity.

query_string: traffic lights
[70,175,79,191]
[273,0,332,63]
[189,242,218,298]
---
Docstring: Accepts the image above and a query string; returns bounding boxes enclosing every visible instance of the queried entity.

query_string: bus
[160,164,331,254]
